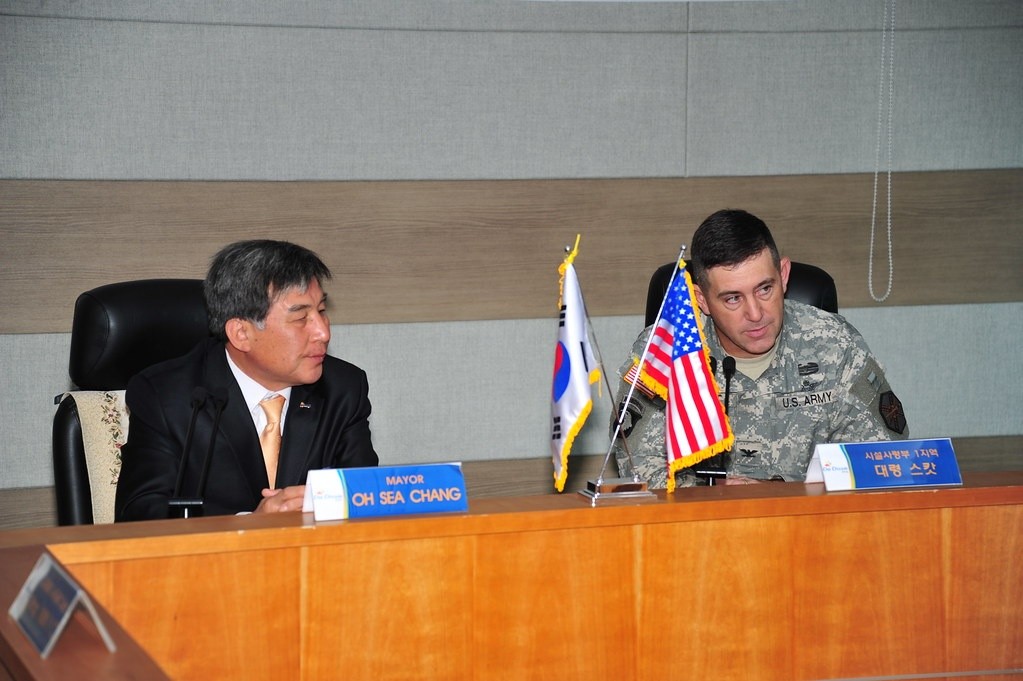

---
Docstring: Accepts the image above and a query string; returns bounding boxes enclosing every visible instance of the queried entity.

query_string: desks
[1,436,1023,681]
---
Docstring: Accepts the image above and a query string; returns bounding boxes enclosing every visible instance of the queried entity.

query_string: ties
[259,394,285,490]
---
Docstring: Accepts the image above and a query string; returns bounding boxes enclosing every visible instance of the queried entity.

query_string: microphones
[168,386,231,520]
[694,356,736,487]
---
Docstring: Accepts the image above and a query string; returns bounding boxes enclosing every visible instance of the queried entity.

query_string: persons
[113,239,380,524]
[609,209,910,490]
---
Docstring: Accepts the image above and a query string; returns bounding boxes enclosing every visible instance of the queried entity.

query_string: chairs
[52,279,207,526]
[645,263,838,328]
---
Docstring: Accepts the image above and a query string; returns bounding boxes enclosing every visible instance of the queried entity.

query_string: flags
[551,261,605,492]
[640,261,736,494]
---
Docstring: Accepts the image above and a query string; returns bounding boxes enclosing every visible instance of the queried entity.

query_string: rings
[745,480,748,484]
